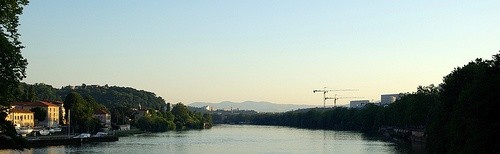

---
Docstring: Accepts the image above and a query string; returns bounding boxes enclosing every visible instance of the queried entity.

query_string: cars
[12,123,109,139]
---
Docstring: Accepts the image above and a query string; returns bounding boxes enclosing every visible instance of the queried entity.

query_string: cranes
[324,94,369,108]
[312,86,359,108]
